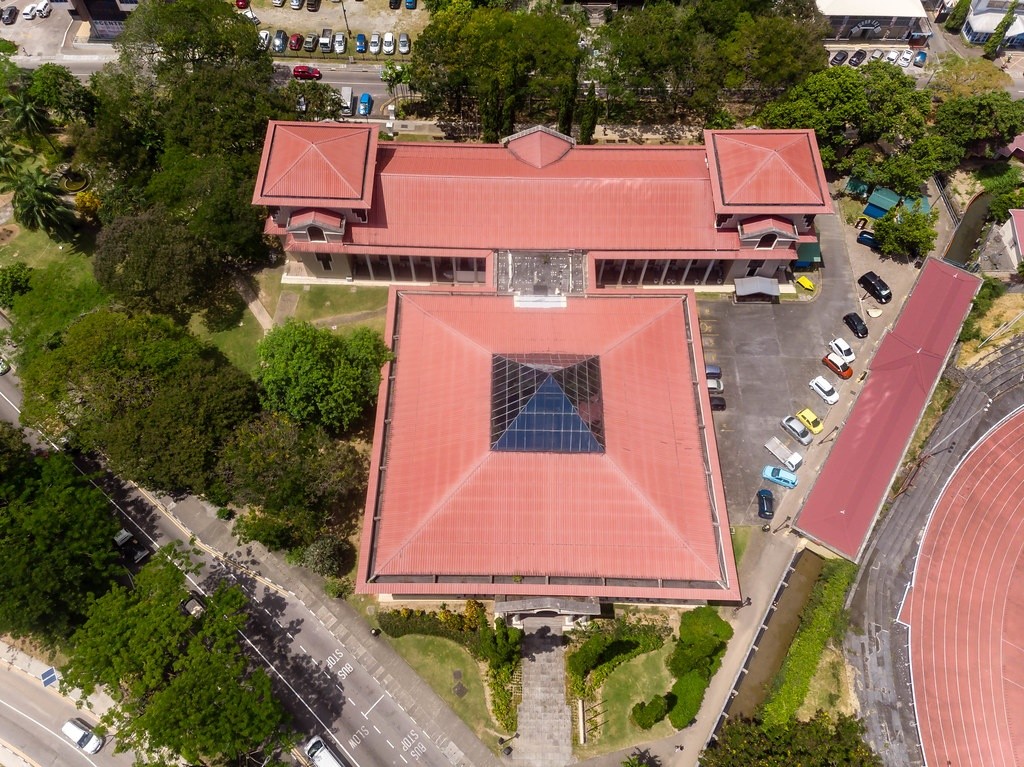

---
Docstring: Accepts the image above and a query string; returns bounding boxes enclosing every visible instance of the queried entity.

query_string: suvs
[61,718,103,755]
[303,737,344,767]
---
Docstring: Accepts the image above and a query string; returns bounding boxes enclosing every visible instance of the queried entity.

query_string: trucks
[764,436,803,472]
[113,528,149,564]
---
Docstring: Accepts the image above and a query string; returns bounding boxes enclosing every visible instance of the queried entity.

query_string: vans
[858,271,893,304]
[1,1,51,24]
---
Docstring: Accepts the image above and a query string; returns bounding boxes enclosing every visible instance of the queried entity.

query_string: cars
[843,313,869,338]
[181,596,207,620]
[822,353,853,380]
[757,490,774,519]
[0,358,10,377]
[763,466,799,489]
[808,375,840,406]
[235,0,417,116]
[706,365,726,411]
[831,49,927,70]
[829,337,856,365]
[796,408,824,435]
[781,416,814,446]
